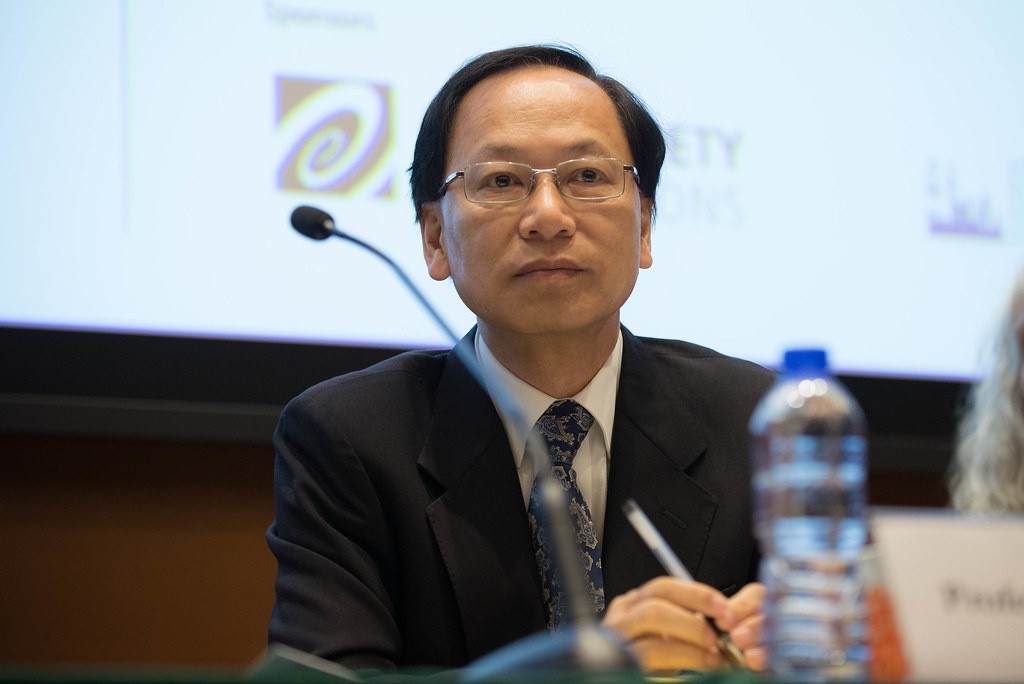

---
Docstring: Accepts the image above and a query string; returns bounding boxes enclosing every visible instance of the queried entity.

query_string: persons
[262,44,777,677]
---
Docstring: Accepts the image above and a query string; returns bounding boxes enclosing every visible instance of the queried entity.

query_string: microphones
[291,203,638,675]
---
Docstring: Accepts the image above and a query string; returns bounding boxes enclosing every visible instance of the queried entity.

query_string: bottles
[748,348,872,680]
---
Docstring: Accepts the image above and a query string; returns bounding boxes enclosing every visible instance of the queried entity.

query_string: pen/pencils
[623,498,744,663]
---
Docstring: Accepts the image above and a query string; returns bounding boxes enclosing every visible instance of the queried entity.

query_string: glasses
[438,157,641,204]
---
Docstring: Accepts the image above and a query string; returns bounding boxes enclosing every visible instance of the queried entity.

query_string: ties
[528,398,605,643]
